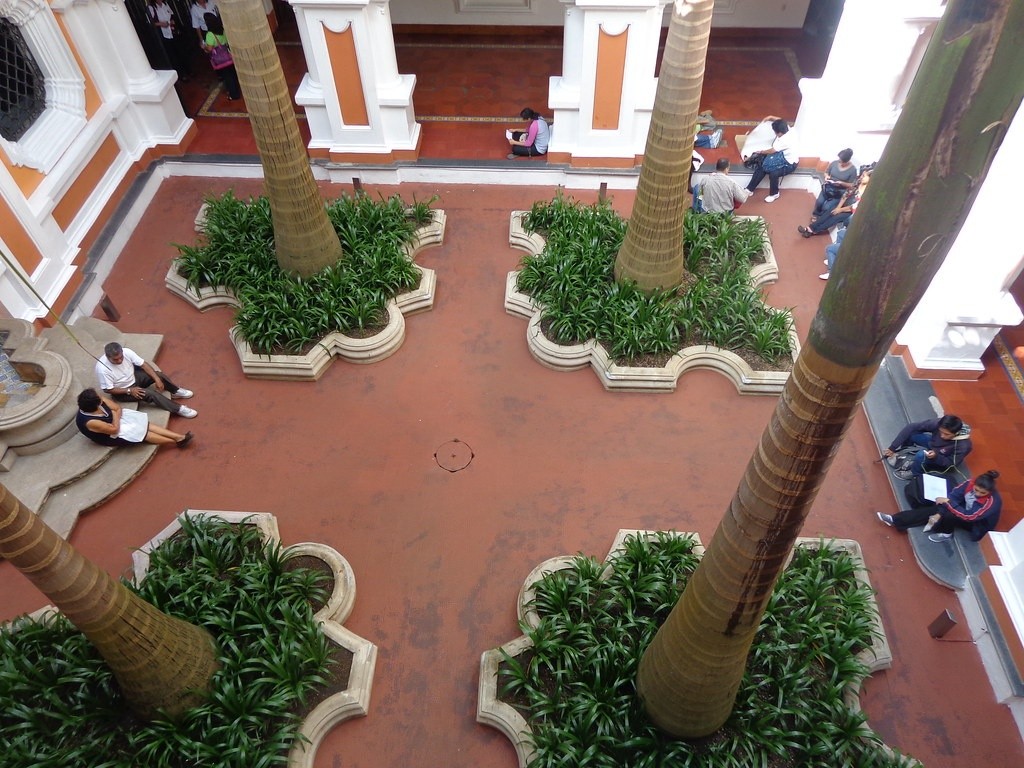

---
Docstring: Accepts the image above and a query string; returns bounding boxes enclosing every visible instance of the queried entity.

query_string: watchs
[127,388,131,396]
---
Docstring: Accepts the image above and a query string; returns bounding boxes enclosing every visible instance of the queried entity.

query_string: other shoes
[764,191,779,202]
[799,226,812,237]
[508,153,519,159]
[177,431,194,447]
[700,110,713,116]
[819,272,830,280]
[824,259,828,266]
[712,129,722,148]
[745,188,753,196]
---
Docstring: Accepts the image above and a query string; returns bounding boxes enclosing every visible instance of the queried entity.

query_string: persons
[691,157,750,218]
[883,414,971,480]
[693,109,723,148]
[143,0,221,72]
[96,342,198,419]
[76,390,195,449]
[799,146,877,280]
[506,107,549,159]
[875,469,1003,542]
[201,13,241,100]
[742,114,800,204]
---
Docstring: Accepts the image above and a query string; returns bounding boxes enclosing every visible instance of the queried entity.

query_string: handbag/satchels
[744,152,766,169]
[110,408,148,442]
[886,446,925,469]
[762,152,788,173]
[905,475,933,509]
[822,183,840,200]
[210,47,233,71]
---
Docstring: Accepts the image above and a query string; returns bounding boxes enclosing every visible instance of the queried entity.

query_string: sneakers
[172,387,193,399]
[877,511,893,527]
[177,405,198,418]
[929,533,952,542]
[893,470,913,480]
